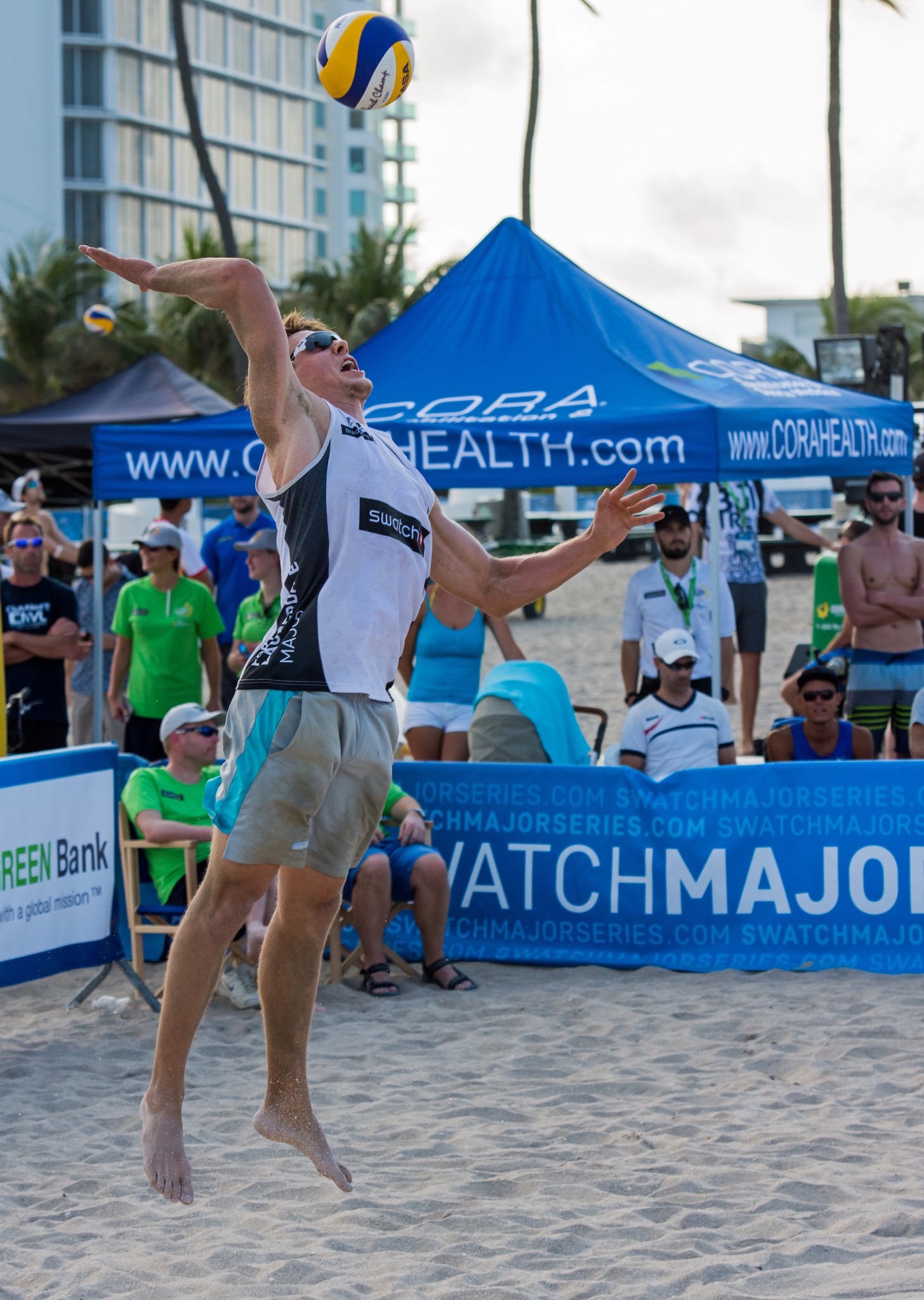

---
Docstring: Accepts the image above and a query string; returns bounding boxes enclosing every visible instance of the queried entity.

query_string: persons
[399,570,525,763]
[334,774,478,998]
[622,627,736,782]
[687,473,844,755]
[623,504,738,710]
[0,470,287,762]
[122,703,270,1009]
[778,441,924,760]
[68,238,669,1204]
[765,667,875,764]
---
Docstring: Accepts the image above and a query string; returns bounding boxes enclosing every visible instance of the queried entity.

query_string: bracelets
[51,544,64,559]
[624,690,638,702]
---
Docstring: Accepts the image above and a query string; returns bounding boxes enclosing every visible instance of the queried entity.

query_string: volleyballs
[81,302,118,340]
[314,9,415,111]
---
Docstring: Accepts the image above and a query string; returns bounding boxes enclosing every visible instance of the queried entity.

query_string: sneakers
[216,965,262,1011]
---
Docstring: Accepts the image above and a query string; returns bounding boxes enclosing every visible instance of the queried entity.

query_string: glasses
[81,574,92,581]
[869,490,902,502]
[3,538,45,548]
[27,480,37,488]
[174,725,219,738]
[290,331,341,363]
[660,659,696,671]
[802,688,838,702]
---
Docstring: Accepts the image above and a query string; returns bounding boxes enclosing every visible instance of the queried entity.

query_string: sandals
[358,963,400,997]
[421,955,477,992]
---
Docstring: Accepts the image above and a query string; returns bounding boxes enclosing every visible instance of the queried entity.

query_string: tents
[87,213,915,752]
[0,361,244,471]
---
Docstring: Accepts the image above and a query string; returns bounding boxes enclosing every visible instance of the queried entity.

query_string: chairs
[118,802,256,999]
[325,820,434,983]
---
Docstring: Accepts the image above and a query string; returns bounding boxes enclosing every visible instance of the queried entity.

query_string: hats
[159,702,223,743]
[655,628,701,666]
[233,528,277,552]
[11,469,40,501]
[796,665,839,689]
[655,505,690,532]
[131,520,182,550]
